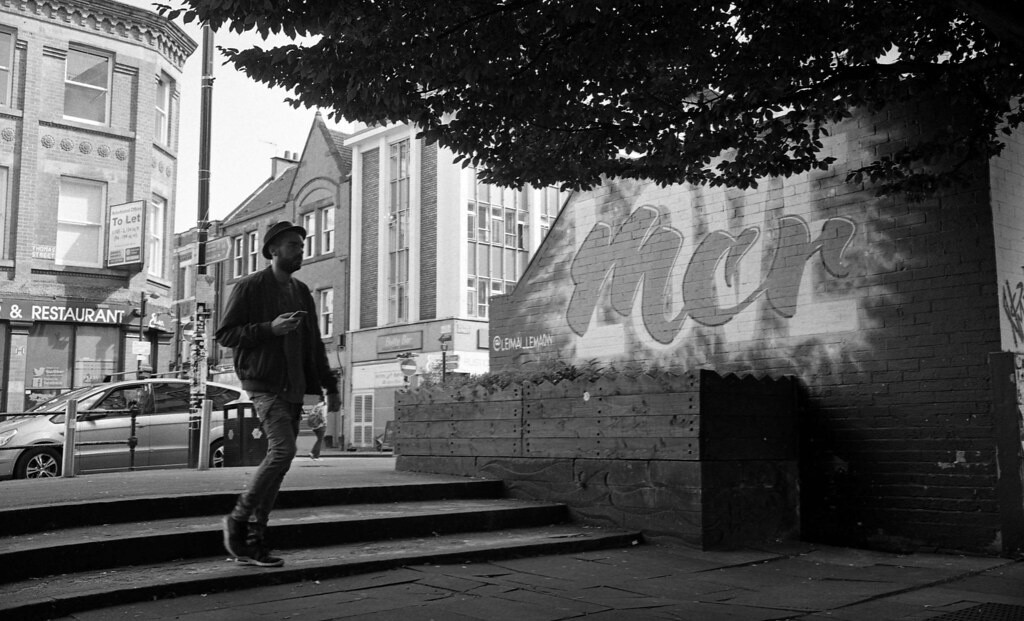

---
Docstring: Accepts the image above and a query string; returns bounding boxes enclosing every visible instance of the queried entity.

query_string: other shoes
[309,453,314,458]
[313,458,324,461]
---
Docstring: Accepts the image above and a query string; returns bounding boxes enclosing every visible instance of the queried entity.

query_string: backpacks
[308,404,325,428]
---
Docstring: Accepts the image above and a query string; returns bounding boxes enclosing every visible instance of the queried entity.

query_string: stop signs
[401,359,417,375]
[183,321,196,342]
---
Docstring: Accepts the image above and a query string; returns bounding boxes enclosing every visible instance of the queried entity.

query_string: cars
[0,378,264,480]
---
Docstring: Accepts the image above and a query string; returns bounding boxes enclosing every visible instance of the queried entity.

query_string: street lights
[136,292,160,375]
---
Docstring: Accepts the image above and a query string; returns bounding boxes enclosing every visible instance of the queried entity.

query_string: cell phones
[289,311,307,318]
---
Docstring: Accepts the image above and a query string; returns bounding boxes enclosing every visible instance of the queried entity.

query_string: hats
[261,221,306,260]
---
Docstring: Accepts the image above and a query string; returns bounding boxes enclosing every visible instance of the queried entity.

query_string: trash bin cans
[223,399,268,468]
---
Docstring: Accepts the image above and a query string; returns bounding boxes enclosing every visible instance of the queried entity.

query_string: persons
[307,401,328,457]
[136,389,153,414]
[215,221,339,567]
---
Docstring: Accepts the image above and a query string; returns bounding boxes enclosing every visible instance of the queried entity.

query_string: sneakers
[235,541,285,566]
[223,517,248,558]
